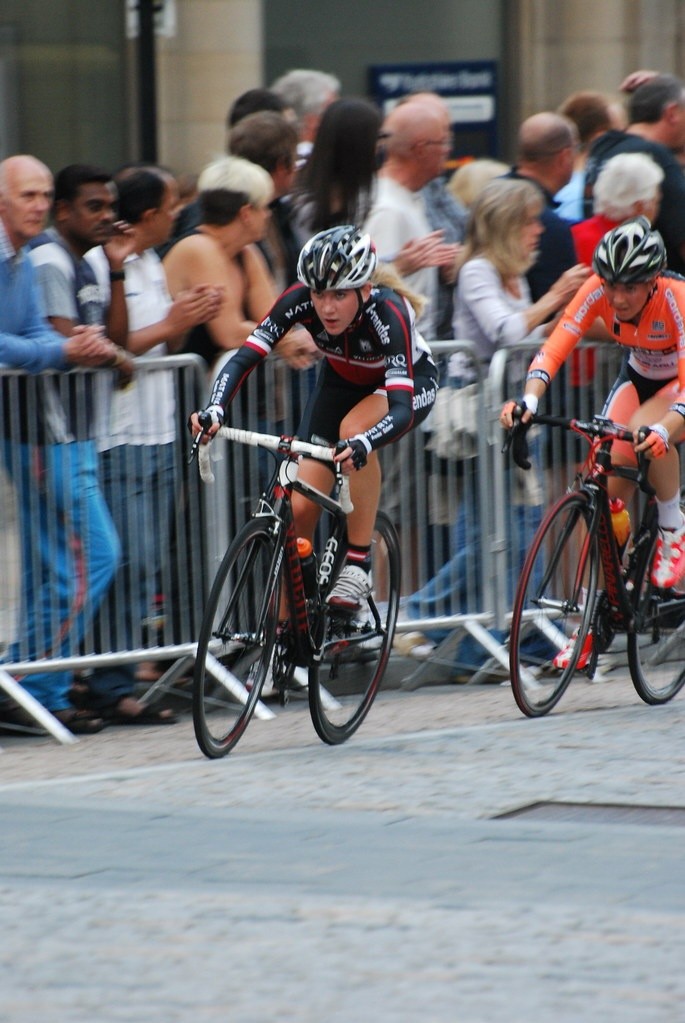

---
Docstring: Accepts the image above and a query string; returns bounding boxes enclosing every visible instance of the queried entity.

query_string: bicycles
[508,397,685,717]
[185,409,404,759]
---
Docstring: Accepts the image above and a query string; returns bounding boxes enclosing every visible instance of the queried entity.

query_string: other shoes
[393,631,438,657]
[0,706,50,736]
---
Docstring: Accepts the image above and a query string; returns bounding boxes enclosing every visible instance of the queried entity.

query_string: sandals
[90,693,180,725]
[51,706,111,735]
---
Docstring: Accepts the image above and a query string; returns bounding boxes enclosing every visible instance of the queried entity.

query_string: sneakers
[245,642,287,697]
[323,564,374,613]
[551,627,592,673]
[650,510,685,589]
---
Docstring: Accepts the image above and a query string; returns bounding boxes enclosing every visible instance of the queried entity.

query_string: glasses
[376,131,392,141]
[409,135,450,151]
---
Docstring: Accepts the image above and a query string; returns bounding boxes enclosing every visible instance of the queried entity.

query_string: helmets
[295,224,378,291]
[592,215,668,284]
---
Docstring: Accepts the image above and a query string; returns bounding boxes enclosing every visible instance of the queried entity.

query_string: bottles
[296,539,319,599]
[607,495,634,552]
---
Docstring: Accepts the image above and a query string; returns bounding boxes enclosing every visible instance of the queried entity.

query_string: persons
[500,215,685,671]
[0,68,685,735]
[189,224,438,694]
